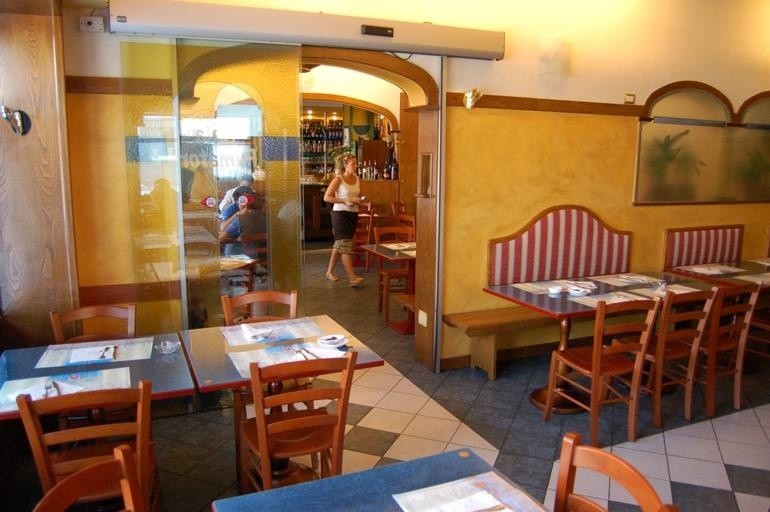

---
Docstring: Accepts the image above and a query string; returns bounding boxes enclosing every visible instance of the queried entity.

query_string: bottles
[302,120,345,183]
[357,158,398,180]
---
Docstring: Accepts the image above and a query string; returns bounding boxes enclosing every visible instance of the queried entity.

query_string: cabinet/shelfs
[300,125,343,167]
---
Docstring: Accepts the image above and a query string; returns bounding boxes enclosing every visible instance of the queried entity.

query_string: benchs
[442,305,677,379]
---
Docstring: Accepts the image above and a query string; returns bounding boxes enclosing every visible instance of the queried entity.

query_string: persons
[218,174,267,261]
[324,153,369,287]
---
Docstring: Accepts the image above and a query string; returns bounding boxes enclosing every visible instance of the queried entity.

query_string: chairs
[353,206,416,334]
[235,350,358,494]
[16,378,160,512]
[542,295,664,448]
[221,289,314,419]
[49,303,137,451]
[221,227,270,275]
[614,281,769,428]
[553,432,678,512]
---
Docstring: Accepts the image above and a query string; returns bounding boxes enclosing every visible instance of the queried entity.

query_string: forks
[99,347,109,359]
[44,382,52,398]
[291,344,308,360]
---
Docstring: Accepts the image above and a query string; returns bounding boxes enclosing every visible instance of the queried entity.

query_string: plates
[154,341,182,355]
[547,285,592,298]
[392,243,412,250]
[316,334,349,348]
[617,274,641,283]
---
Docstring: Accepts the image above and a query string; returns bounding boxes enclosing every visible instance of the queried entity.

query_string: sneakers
[347,275,365,287]
[325,271,339,282]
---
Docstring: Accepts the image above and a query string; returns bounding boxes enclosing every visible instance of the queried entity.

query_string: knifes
[52,381,61,396]
[112,345,119,360]
[302,347,318,359]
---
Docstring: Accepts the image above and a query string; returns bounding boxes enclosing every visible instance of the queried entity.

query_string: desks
[0,314,196,498]
[132,208,221,220]
[137,223,219,252]
[483,261,736,414]
[178,314,385,494]
[213,446,551,512]
[663,258,769,379]
[146,254,255,292]
